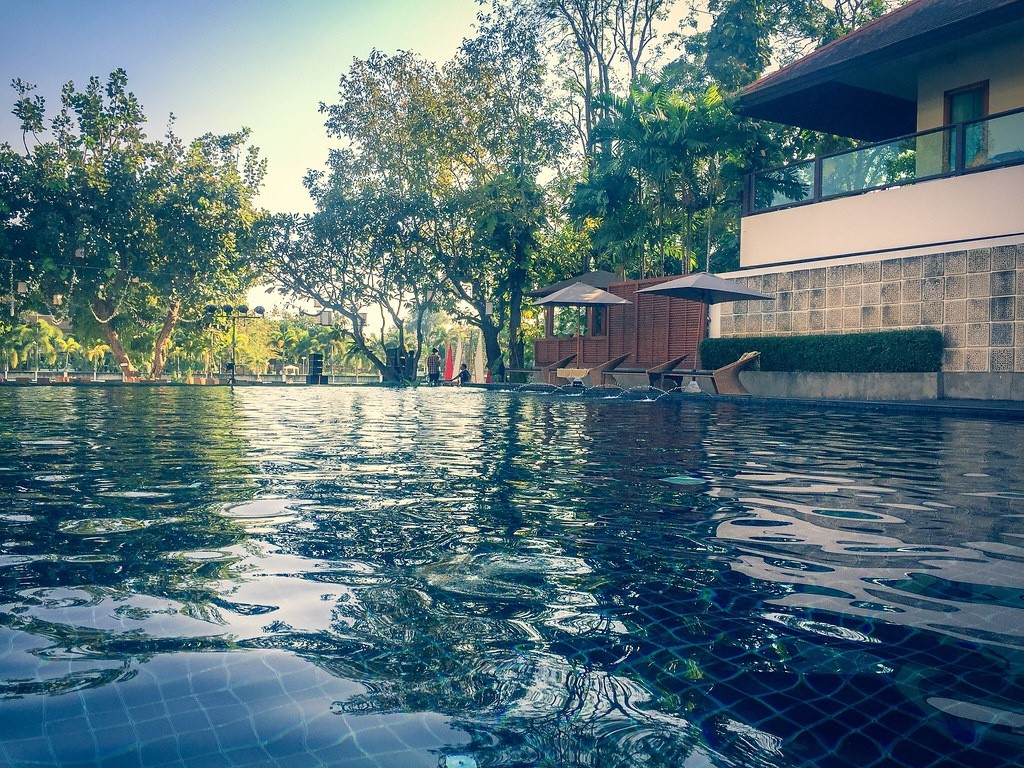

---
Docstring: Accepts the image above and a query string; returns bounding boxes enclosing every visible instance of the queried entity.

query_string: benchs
[505,351,763,395]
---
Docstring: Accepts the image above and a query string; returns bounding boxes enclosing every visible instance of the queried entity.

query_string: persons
[427,348,440,381]
[408,351,418,376]
[451,363,471,383]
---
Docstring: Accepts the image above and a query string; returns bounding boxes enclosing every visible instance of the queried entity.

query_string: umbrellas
[634,271,776,372]
[529,280,633,368]
